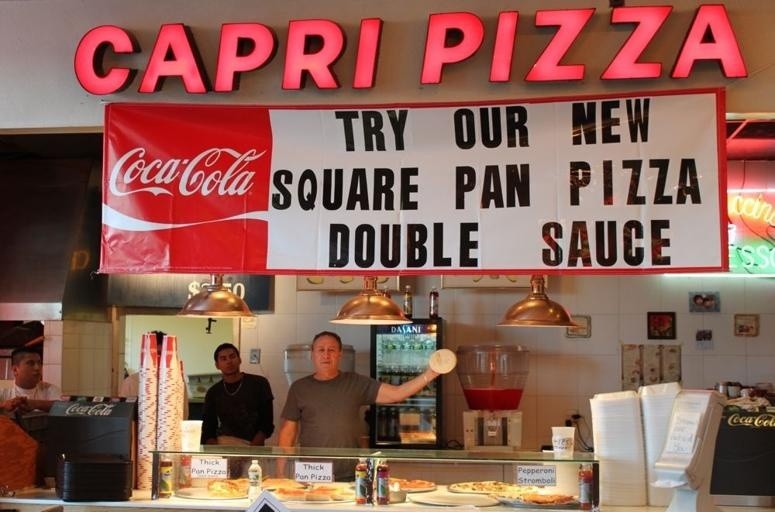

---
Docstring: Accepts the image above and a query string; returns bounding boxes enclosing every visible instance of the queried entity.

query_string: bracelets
[421,373,429,385]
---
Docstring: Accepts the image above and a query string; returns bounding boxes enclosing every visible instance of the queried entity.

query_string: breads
[206,477,356,500]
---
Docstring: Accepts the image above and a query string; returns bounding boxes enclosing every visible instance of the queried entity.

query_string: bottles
[403,286,414,317]
[382,285,391,299]
[715,380,773,401]
[248,459,270,501]
[579,462,593,509]
[429,286,439,317]
[376,335,436,439]
[355,457,390,506]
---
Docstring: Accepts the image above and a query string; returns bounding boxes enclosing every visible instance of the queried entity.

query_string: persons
[1,348,64,411]
[275,332,439,482]
[121,332,190,421]
[200,343,274,478]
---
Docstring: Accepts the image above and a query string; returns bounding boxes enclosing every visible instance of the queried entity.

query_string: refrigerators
[367,317,445,446]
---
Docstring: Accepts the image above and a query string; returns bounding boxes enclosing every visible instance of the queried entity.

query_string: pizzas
[374,479,436,492]
[448,480,576,504]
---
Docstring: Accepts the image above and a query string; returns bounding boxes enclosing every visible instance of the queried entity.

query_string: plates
[427,348,458,374]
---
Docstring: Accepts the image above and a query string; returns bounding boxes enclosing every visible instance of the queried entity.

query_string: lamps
[177,273,256,319]
[329,276,413,326]
[496,275,578,327]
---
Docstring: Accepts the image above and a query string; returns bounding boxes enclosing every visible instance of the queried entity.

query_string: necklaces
[222,374,244,395]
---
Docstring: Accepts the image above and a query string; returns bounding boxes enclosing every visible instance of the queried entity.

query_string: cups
[550,425,576,458]
[131,331,185,497]
[181,420,206,452]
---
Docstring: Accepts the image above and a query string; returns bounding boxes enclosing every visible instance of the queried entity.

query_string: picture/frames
[566,314,591,337]
[647,312,677,339]
[734,313,759,336]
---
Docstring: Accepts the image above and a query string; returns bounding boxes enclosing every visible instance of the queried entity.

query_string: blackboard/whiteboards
[0,128,275,315]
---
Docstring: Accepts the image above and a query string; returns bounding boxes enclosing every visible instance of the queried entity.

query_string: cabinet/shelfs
[149,444,600,512]
